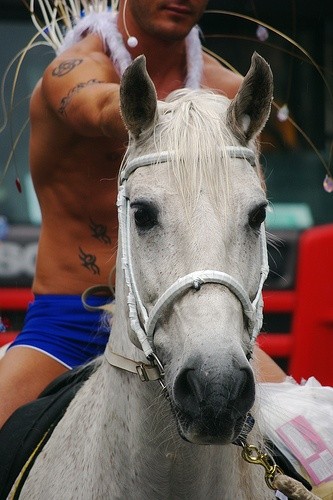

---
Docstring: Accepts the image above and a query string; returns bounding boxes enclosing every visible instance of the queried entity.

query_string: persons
[0,0,301,429]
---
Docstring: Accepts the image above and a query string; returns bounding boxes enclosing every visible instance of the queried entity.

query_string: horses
[0,51,298,499]
